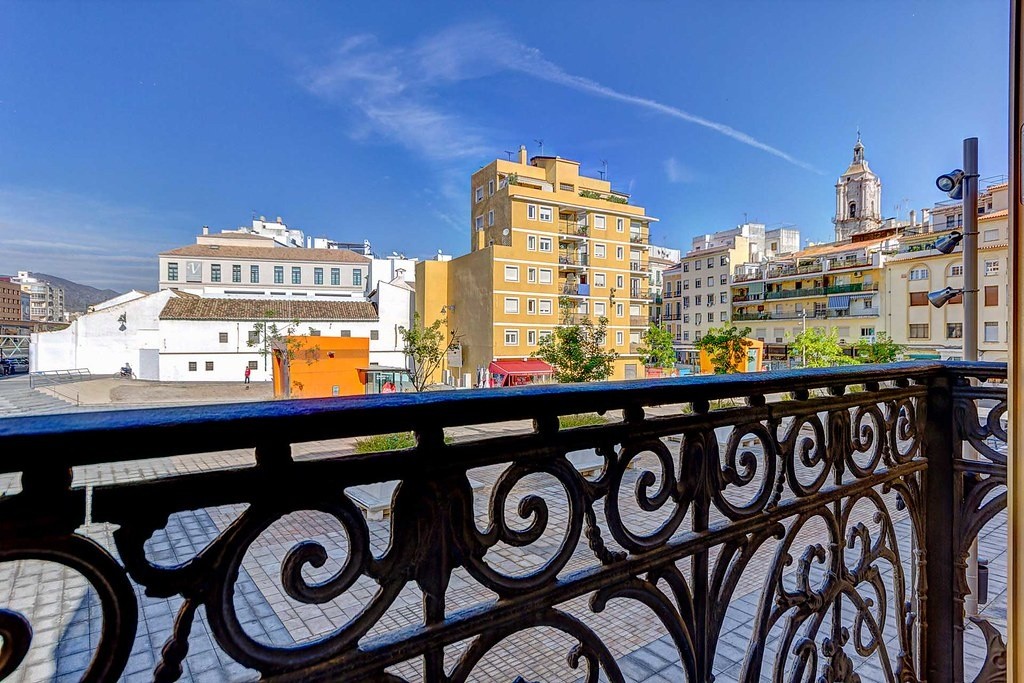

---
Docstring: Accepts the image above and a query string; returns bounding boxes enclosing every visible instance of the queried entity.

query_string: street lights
[925,137,981,457]
[798,308,806,367]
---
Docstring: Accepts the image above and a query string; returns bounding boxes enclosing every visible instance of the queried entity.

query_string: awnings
[828,295,874,309]
[488,359,556,387]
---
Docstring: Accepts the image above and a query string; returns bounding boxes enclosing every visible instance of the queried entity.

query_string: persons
[126,363,131,369]
[245,366,250,384]
[762,364,768,372]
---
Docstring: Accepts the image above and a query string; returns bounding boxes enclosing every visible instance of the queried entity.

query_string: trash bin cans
[4,367,9,375]
[11,366,15,375]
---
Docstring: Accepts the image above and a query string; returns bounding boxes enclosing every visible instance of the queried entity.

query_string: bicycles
[113,368,137,381]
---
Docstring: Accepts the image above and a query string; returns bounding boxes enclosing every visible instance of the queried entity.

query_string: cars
[0,360,28,376]
